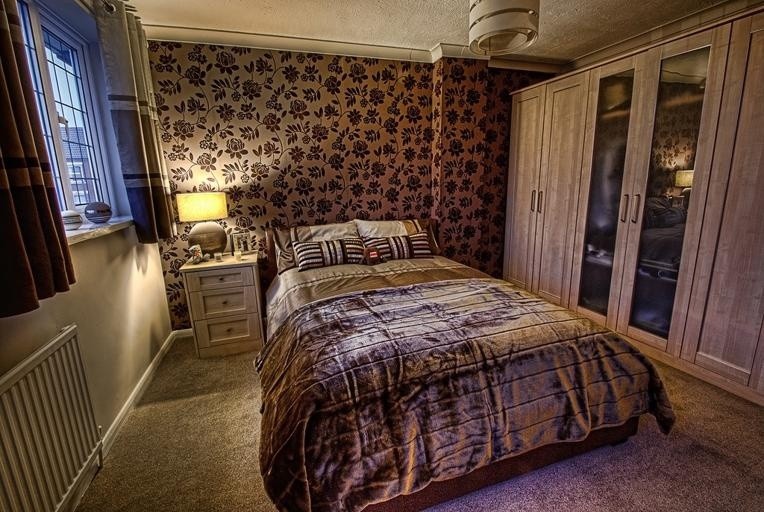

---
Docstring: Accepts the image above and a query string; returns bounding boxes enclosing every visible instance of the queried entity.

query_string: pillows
[272,215,442,275]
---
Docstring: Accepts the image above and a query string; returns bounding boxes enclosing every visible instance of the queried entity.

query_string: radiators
[1,321,104,511]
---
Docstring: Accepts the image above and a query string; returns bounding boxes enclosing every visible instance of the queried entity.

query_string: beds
[262,217,650,512]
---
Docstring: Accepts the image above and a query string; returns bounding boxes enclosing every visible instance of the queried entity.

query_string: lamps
[675,169,694,187]
[466,1,541,58]
[175,190,228,255]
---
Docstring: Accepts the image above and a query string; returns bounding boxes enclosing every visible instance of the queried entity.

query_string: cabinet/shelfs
[179,249,267,361]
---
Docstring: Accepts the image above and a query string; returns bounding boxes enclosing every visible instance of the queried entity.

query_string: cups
[213,251,242,262]
